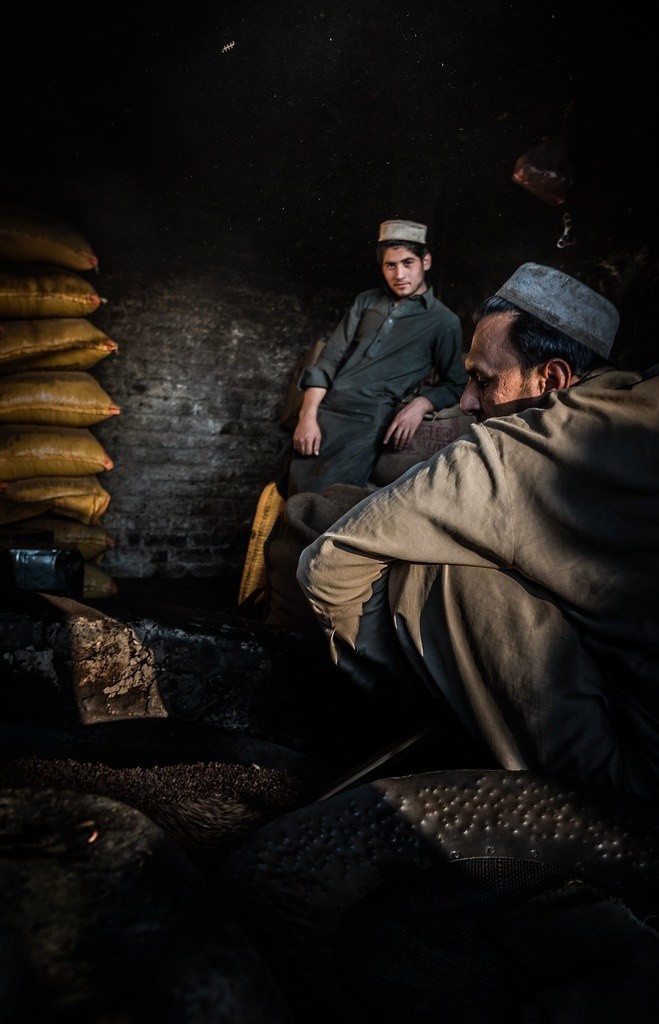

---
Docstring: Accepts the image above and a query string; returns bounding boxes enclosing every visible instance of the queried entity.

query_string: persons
[294,259,658,822]
[236,218,468,609]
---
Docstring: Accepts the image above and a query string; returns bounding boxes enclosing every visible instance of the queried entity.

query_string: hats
[494,262,619,359]
[378,219,428,245]
